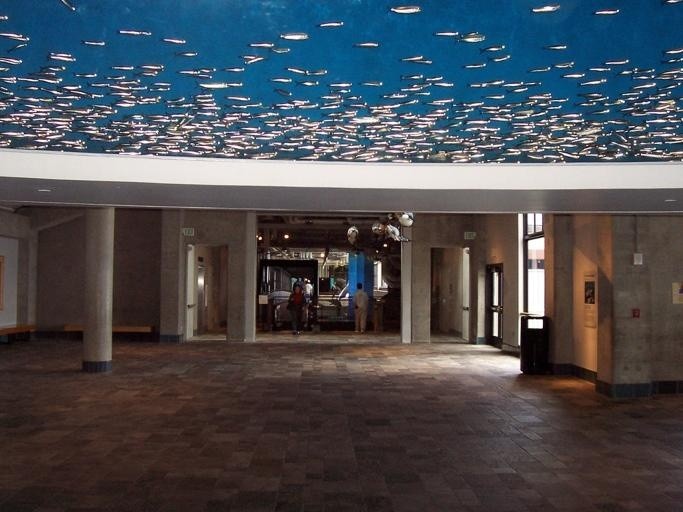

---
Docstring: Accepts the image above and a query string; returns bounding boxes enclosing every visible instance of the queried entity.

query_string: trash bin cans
[521,316,549,375]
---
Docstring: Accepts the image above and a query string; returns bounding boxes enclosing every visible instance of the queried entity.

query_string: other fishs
[1,1,683,164]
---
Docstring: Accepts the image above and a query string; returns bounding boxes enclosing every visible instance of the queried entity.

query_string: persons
[287,278,314,335]
[353,283,369,334]
